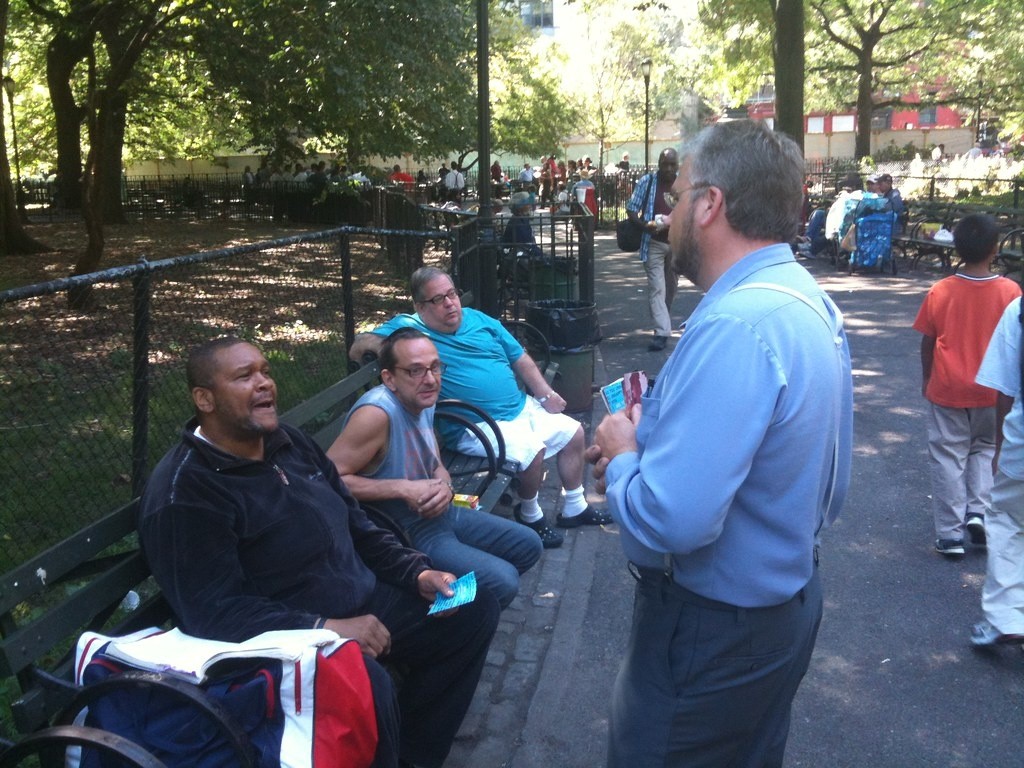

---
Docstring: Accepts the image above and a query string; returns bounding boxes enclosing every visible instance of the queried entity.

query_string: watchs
[438,480,456,503]
[537,391,557,403]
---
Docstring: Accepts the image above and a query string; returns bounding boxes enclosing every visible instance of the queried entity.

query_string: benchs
[893,199,1024,285]
[1,292,563,768]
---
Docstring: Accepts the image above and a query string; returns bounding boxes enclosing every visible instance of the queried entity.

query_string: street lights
[640,60,654,174]
[2,76,28,215]
[976,69,985,144]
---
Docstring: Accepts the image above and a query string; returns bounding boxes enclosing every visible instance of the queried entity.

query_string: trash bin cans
[523,297,596,413]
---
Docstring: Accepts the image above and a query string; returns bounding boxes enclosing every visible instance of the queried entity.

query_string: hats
[508,192,530,213]
[866,175,878,184]
[874,174,892,183]
[579,169,589,179]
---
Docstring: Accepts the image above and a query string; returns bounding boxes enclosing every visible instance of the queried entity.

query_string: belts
[664,580,803,615]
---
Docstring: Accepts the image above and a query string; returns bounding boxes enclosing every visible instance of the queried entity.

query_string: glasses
[394,363,447,378]
[663,187,690,208]
[421,288,458,304]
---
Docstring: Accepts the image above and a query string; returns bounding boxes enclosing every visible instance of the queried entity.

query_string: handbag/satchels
[617,218,641,252]
[65,627,378,768]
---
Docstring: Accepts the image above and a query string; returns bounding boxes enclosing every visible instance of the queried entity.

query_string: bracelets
[316,616,328,629]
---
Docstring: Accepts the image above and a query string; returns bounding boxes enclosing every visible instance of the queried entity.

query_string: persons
[349,266,615,550]
[911,213,1023,554]
[437,187,547,268]
[624,147,681,351]
[931,143,945,165]
[136,336,502,768]
[967,282,1024,648]
[959,138,1024,164]
[324,326,544,613]
[584,118,854,768]
[796,172,905,274]
[491,151,632,231]
[241,148,469,224]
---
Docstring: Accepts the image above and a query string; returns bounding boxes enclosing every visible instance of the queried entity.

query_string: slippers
[513,503,564,549]
[556,504,613,528]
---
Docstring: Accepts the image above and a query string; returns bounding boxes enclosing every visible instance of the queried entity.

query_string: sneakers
[797,242,815,259]
[648,336,667,350]
[966,513,986,544]
[970,619,1024,646]
[796,234,810,243]
[936,539,964,554]
